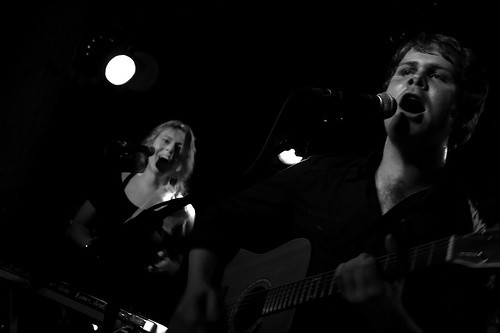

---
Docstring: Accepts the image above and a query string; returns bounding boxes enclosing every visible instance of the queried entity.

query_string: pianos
[0,262,169,333]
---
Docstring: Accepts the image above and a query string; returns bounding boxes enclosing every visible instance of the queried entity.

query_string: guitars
[168,226,497,333]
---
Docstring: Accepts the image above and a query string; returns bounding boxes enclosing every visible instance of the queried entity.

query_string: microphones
[118,140,155,157]
[324,88,397,119]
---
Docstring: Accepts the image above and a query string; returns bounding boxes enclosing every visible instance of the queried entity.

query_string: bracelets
[80,240,91,248]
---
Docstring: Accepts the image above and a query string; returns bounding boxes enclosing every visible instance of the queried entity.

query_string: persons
[169,31,500,333]
[63,121,197,315]
